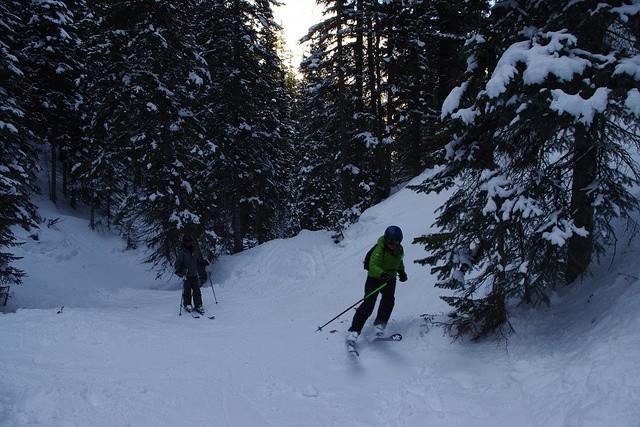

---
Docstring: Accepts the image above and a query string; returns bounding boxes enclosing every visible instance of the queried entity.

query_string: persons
[346,225,408,346]
[175,233,207,313]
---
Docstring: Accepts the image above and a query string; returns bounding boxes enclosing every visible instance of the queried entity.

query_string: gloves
[399,273,407,282]
[381,273,390,281]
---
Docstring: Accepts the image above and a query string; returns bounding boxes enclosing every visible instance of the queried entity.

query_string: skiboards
[179,303,215,320]
[346,333,402,356]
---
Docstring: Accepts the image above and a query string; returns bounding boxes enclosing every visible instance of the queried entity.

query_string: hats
[183,234,193,243]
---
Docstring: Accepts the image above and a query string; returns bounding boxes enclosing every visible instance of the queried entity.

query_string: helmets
[384,226,403,248]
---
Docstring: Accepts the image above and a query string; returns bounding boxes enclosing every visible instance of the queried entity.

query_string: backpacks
[364,243,385,271]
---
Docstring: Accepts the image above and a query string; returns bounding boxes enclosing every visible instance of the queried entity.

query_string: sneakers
[196,305,204,311]
[347,331,357,342]
[373,326,384,335]
[184,304,193,312]
